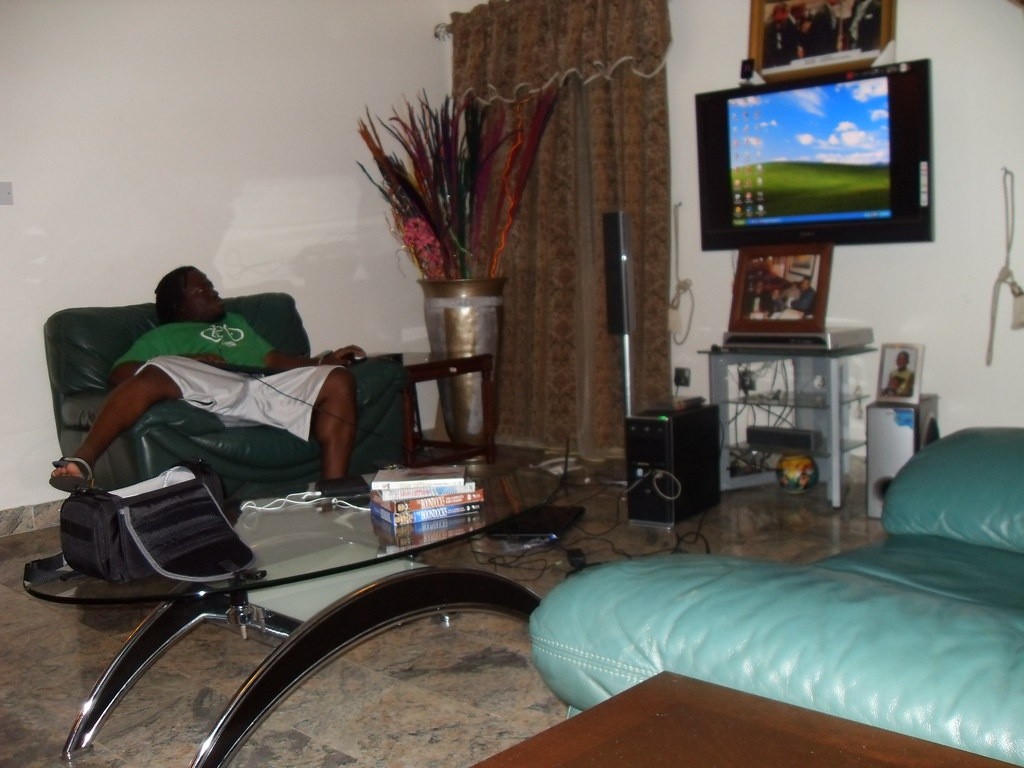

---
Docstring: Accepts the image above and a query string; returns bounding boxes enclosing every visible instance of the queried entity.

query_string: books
[370,466,484,528]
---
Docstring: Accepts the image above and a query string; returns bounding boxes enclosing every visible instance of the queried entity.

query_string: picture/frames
[740,0,896,87]
[875,341,925,405]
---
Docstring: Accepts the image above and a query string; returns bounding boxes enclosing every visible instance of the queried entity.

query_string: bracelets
[319,355,322,366]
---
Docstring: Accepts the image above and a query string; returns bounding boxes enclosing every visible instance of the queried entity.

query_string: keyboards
[197,360,289,376]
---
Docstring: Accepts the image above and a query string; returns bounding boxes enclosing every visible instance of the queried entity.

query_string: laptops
[485,505,584,540]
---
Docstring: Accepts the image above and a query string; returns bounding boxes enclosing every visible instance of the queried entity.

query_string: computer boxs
[625,404,720,531]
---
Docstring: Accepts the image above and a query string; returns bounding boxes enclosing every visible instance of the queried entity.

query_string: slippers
[50,458,94,493]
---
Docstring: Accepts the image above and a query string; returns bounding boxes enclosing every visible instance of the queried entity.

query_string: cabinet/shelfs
[694,346,877,507]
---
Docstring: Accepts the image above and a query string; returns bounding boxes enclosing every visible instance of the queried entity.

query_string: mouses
[341,352,367,362]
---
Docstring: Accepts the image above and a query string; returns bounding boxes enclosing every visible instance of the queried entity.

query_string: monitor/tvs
[693,58,935,251]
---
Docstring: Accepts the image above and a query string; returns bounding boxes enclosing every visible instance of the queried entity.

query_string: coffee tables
[23,467,566,768]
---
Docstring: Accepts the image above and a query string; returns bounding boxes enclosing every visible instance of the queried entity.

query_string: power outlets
[674,367,691,386]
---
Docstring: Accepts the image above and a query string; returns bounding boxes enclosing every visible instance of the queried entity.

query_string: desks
[396,349,494,471]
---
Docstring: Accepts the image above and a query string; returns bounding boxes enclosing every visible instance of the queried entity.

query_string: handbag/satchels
[59,460,255,583]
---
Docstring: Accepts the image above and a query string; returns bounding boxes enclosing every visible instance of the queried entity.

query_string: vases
[414,274,508,463]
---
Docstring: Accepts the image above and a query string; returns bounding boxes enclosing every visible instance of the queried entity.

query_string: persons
[885,351,915,398]
[49,266,366,492]
[762,0,882,66]
[749,276,813,318]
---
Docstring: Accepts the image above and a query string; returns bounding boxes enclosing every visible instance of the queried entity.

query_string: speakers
[603,211,636,335]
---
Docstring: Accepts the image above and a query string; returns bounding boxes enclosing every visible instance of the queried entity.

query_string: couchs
[43,290,405,510]
[529,425,1023,768]
